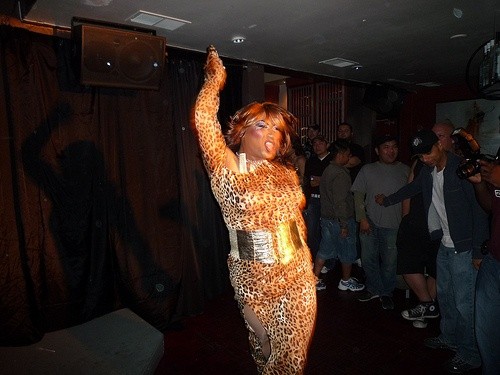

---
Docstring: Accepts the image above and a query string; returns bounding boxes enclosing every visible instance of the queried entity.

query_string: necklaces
[235,151,265,167]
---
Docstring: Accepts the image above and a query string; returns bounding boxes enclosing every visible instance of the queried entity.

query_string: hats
[374,133,398,146]
[307,122,321,131]
[311,134,326,142]
[409,128,440,159]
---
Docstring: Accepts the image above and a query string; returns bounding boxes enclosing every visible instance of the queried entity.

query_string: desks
[0,309,165,375]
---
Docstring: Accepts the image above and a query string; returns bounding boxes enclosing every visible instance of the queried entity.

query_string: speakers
[70,24,168,91]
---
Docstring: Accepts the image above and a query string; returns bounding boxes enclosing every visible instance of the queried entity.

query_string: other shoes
[423,334,450,350]
[447,354,479,375]
[320,265,328,274]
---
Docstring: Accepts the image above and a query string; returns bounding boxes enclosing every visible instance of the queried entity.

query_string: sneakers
[337,276,365,291]
[315,282,327,291]
[379,295,395,310]
[357,290,381,302]
[400,301,440,329]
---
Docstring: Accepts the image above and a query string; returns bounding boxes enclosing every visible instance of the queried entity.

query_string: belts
[310,193,321,199]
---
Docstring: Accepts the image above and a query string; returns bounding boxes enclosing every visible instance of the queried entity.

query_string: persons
[288,121,500,375]
[313,140,364,291]
[194,45,319,375]
[374,134,491,375]
[350,135,414,310]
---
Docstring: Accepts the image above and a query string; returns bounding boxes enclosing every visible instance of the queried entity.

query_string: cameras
[455,153,494,180]
